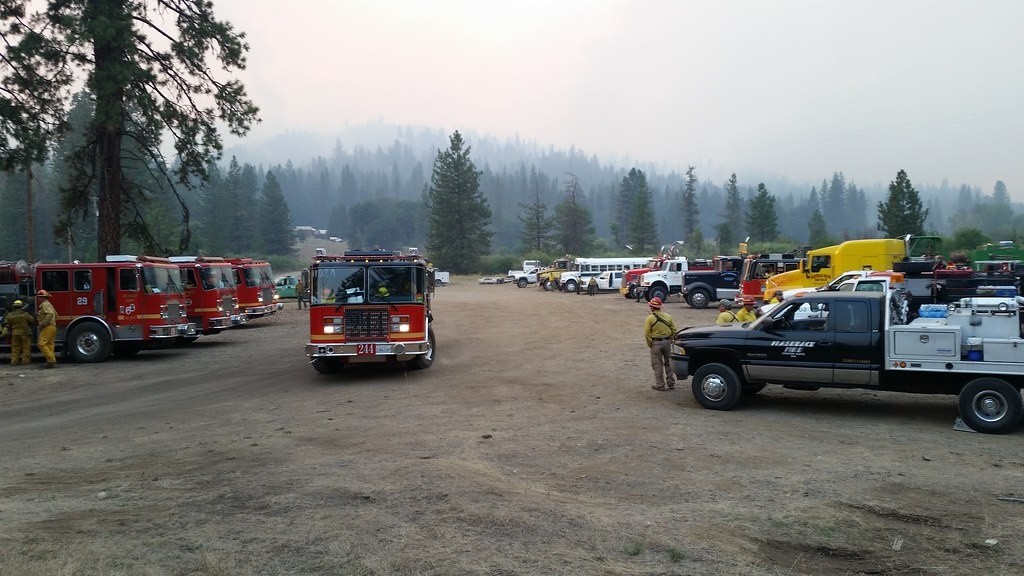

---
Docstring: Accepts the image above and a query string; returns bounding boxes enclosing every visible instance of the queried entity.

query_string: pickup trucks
[508,260,545,278]
[435,271,450,287]
[512,267,546,288]
[669,289,1024,434]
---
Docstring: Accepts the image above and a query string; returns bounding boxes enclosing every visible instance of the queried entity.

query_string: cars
[581,270,622,290]
[274,275,298,298]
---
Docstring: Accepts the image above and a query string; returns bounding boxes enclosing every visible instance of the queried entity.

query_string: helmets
[37,290,49,297]
[13,300,23,306]
[743,295,757,305]
[648,297,663,308]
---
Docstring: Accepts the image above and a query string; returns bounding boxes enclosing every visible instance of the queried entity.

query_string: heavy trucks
[618,233,1024,319]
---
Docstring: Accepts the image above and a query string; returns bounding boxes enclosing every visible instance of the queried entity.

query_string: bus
[536,258,574,291]
[558,257,653,293]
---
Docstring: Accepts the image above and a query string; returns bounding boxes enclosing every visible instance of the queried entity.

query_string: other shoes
[42,361,56,368]
[668,384,674,388]
[652,384,665,390]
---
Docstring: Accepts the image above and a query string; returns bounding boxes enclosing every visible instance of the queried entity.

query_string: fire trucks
[167,255,248,337]
[300,247,435,374]
[223,257,280,320]
[0,255,204,363]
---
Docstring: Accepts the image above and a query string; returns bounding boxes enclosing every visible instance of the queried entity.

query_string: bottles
[920,304,947,317]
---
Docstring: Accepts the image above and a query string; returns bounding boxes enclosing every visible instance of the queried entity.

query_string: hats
[718,299,731,306]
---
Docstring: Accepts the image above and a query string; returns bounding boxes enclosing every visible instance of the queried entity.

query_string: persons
[737,295,757,322]
[644,298,677,392]
[770,290,782,304]
[715,299,740,323]
[576,276,584,295]
[761,267,775,278]
[549,273,555,291]
[589,275,596,296]
[37,289,59,369]
[296,278,307,310]
[0,316,4,338]
[5,300,35,366]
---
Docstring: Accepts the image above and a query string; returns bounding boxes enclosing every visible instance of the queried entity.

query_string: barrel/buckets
[968,350,981,360]
